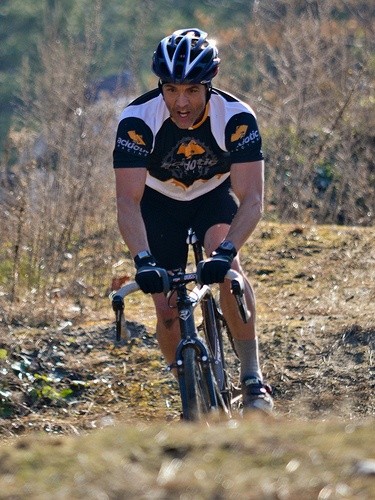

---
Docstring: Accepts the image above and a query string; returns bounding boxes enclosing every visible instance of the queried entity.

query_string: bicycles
[110,228,252,424]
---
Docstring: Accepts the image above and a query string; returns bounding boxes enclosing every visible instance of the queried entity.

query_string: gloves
[133,251,173,296]
[196,240,237,285]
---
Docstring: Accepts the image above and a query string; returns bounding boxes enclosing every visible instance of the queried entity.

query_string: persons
[112,28,274,417]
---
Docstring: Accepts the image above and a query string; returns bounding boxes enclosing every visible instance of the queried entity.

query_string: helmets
[152,28,221,84]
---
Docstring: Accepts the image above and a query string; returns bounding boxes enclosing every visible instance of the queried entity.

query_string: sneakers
[242,374,274,421]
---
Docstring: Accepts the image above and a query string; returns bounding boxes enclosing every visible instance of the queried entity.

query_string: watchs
[219,240,237,257]
